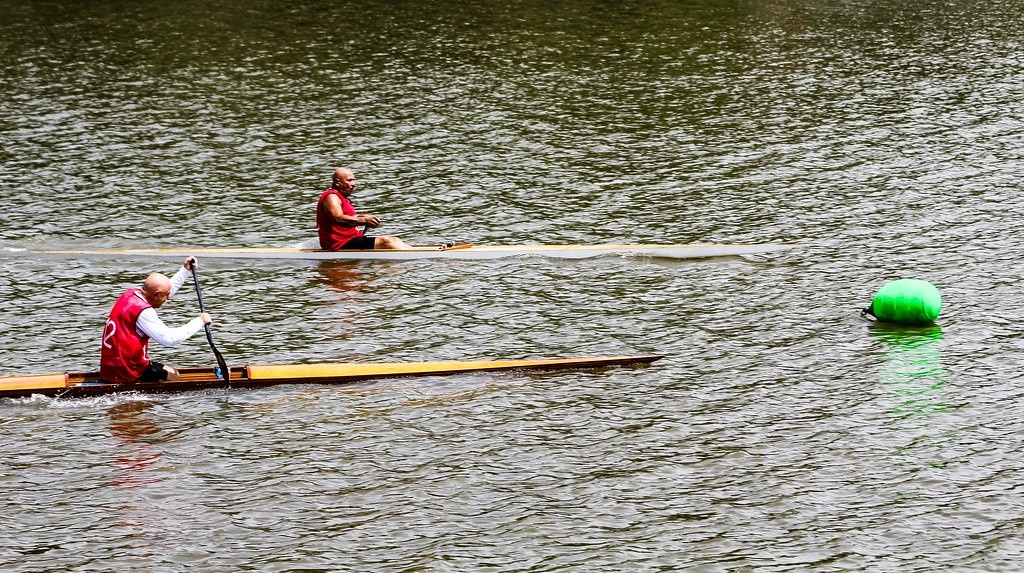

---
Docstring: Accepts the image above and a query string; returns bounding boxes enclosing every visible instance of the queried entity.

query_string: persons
[99,255,212,383]
[316,166,421,251]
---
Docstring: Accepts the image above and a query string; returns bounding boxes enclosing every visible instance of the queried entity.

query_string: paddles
[191,261,230,392]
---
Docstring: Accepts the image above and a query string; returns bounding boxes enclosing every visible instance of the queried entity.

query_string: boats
[0,346,663,398]
[0,225,798,268]
